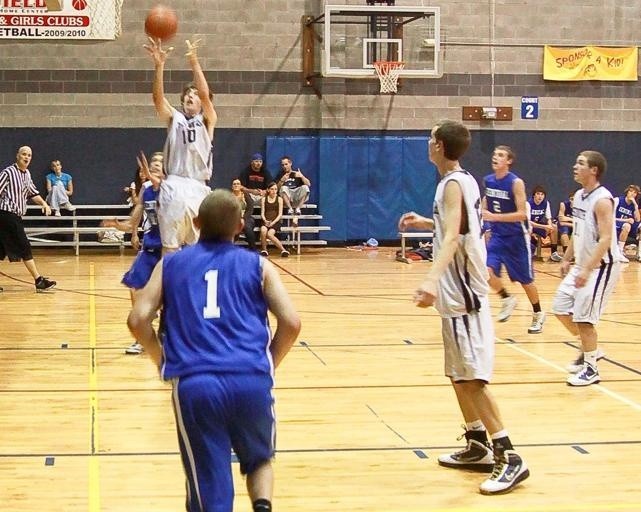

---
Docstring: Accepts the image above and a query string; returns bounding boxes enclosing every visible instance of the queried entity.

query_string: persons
[143,36,218,257]
[528,184,641,268]
[127,188,302,512]
[120,152,175,355]
[398,118,531,496]
[45,159,76,217]
[552,150,621,385]
[123,153,311,257]
[0,145,57,292]
[480,145,546,334]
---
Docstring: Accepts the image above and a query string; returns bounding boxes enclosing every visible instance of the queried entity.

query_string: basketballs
[145,6,178,40]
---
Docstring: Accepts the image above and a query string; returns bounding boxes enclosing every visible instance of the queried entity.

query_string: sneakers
[567,351,604,386]
[126,341,144,354]
[438,432,529,495]
[528,311,544,333]
[550,251,562,262]
[498,295,517,321]
[36,278,56,292]
[288,208,301,215]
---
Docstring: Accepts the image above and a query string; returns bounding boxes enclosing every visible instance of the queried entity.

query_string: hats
[252,154,262,160]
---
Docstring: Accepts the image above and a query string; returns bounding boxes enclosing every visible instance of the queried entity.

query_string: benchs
[398,231,435,259]
[23,201,332,255]
